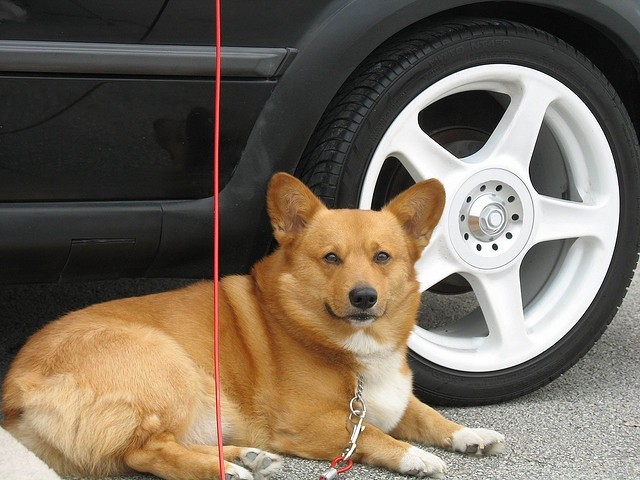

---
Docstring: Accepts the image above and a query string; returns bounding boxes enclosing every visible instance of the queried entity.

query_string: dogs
[1,172,505,480]
